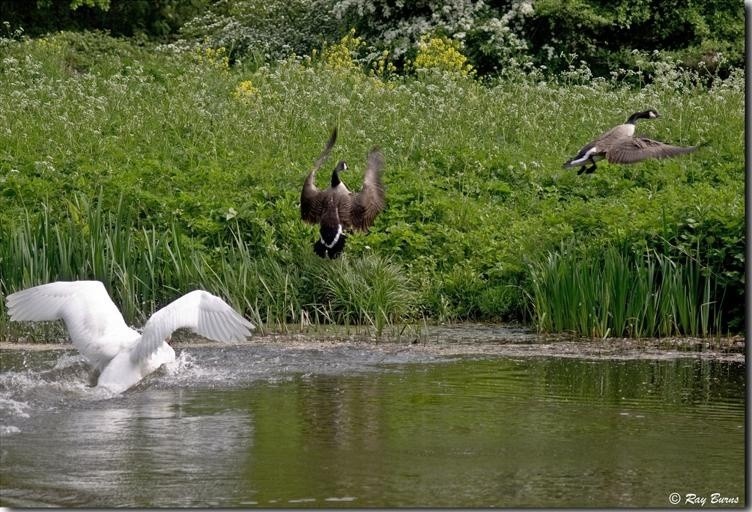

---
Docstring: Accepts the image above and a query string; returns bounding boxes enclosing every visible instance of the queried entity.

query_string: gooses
[5,280,256,397]
[561,109,713,176]
[299,125,385,261]
